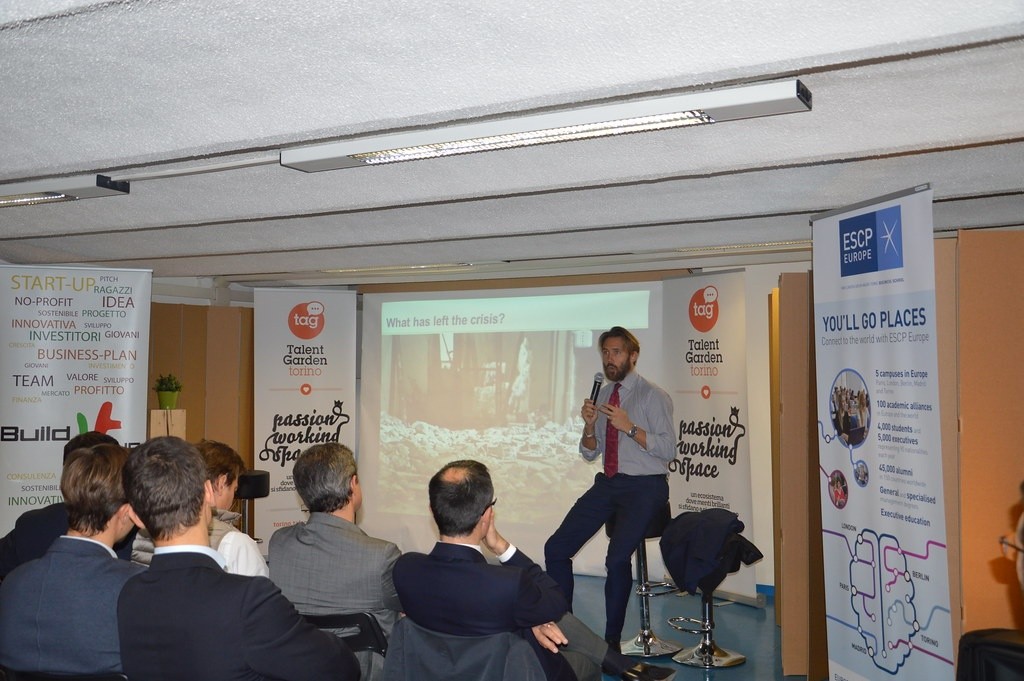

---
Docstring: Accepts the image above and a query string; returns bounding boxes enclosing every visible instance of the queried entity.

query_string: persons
[1000,509,1024,592]
[392,460,675,681]
[0,430,139,584]
[543,326,676,675]
[117,435,363,681]
[830,386,872,509]
[0,444,149,681]
[130,440,270,580]
[268,442,405,681]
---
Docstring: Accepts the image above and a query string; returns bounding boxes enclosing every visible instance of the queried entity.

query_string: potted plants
[156,374,184,409]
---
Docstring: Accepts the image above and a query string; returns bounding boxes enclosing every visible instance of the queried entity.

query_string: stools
[233,470,270,543]
[606,500,684,659]
[659,508,764,668]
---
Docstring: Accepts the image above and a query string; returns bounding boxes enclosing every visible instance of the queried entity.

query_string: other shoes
[602,642,621,676]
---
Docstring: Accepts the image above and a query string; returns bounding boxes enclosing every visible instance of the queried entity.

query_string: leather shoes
[623,663,676,681]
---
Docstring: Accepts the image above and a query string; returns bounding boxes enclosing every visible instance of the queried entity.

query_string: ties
[604,382,622,478]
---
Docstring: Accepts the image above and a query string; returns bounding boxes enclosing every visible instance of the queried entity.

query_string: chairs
[0,665,129,681]
[298,611,387,658]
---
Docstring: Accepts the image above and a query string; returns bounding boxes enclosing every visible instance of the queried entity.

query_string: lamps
[280,79,811,175]
[0,175,130,208]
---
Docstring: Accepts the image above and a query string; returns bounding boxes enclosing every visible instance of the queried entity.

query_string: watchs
[626,424,637,438]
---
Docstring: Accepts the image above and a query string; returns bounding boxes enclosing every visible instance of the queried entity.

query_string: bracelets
[584,430,594,438]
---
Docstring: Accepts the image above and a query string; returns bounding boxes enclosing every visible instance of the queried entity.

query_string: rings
[608,415,610,419]
[547,624,552,627]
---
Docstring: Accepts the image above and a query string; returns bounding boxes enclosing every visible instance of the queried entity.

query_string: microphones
[590,372,605,406]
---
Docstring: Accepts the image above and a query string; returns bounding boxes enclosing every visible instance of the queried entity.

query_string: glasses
[999,535,1024,557]
[481,496,497,517]
